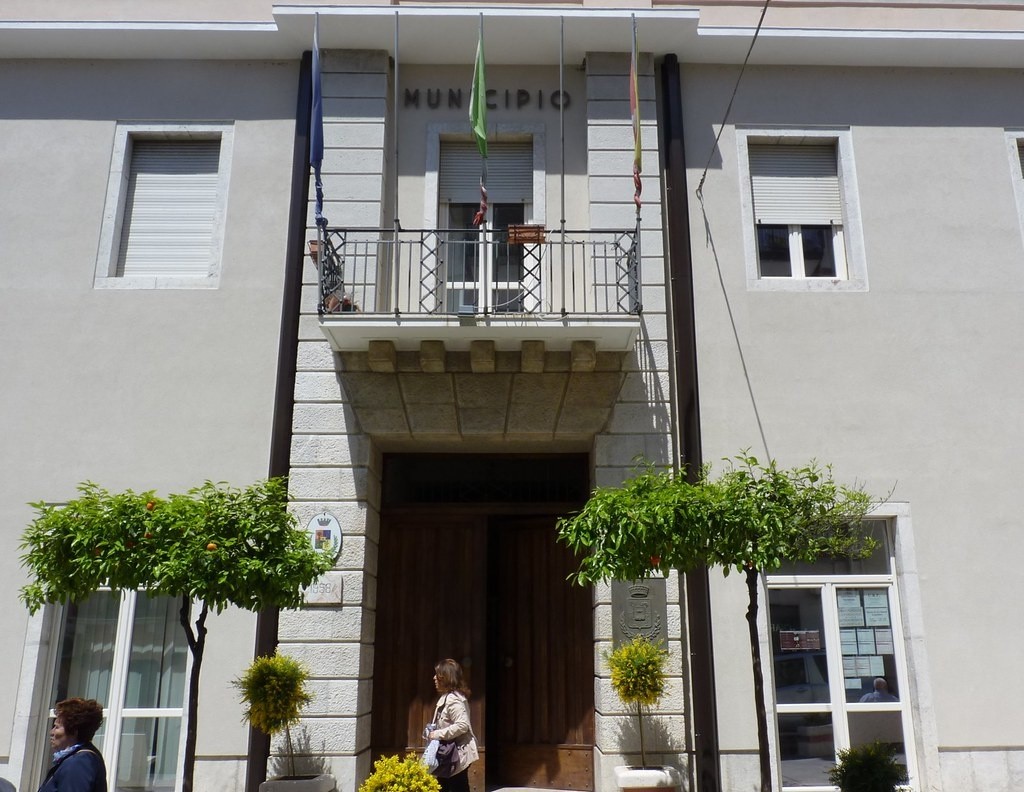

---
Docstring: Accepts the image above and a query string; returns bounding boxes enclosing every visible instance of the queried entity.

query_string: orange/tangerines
[650,555,660,565]
[146,500,156,510]
[144,532,152,539]
[125,540,133,548]
[207,544,218,551]
[94,547,100,555]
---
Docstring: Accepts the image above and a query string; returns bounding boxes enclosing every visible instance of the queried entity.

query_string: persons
[37,696,107,792]
[422,658,479,792]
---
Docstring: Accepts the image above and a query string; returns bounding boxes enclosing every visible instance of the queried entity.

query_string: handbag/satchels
[418,741,438,778]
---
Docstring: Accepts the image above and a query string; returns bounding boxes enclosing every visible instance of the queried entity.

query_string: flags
[629,12,642,207]
[309,13,325,220]
[467,11,490,226]
